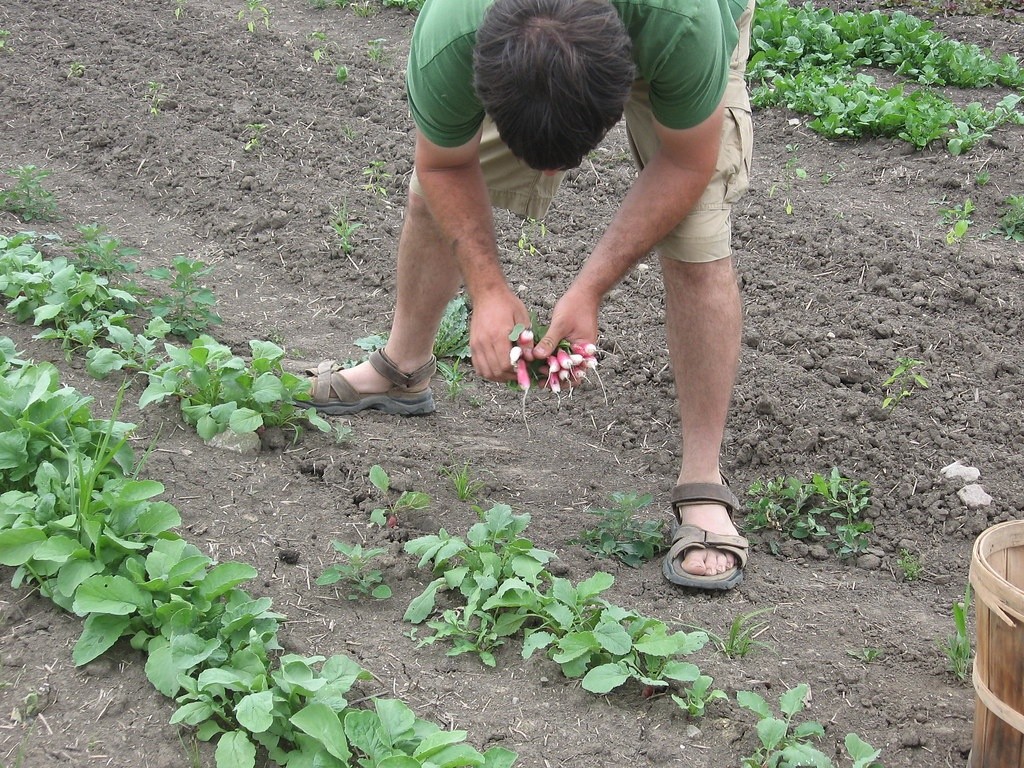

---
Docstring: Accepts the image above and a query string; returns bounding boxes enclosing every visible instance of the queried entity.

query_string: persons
[292,0,755,590]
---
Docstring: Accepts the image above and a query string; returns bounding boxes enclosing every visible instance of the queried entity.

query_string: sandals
[290,346,439,416]
[664,468,751,590]
